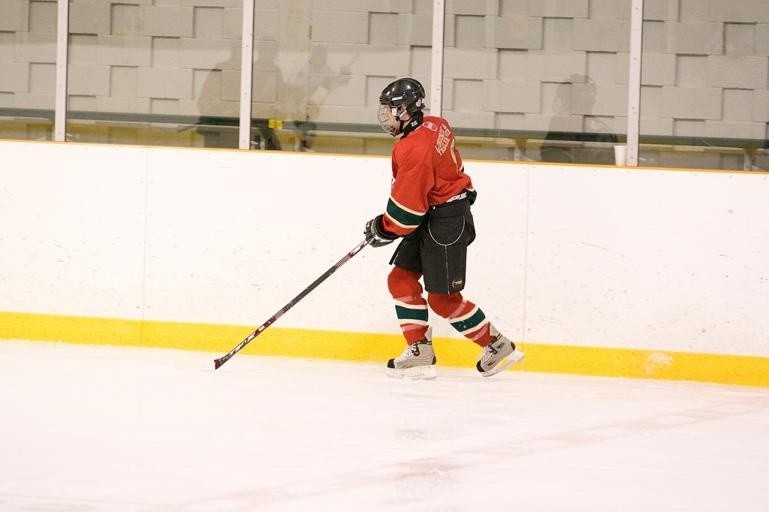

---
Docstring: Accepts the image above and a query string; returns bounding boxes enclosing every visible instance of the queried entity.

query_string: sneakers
[475,321,517,373]
[386,326,438,370]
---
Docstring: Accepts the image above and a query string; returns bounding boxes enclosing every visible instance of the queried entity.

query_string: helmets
[375,77,427,137]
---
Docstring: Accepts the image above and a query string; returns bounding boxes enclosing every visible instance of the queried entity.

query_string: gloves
[363,214,400,248]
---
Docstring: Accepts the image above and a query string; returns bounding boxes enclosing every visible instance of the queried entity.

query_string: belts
[444,191,469,203]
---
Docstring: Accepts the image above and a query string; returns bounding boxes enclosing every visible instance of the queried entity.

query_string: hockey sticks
[181,235,375,375]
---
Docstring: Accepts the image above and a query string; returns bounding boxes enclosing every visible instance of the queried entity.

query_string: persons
[288,46,351,150]
[365,76,523,380]
[538,70,617,166]
[252,36,315,150]
[196,32,241,148]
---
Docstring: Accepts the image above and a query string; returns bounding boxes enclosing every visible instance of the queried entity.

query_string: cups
[613,145,627,166]
